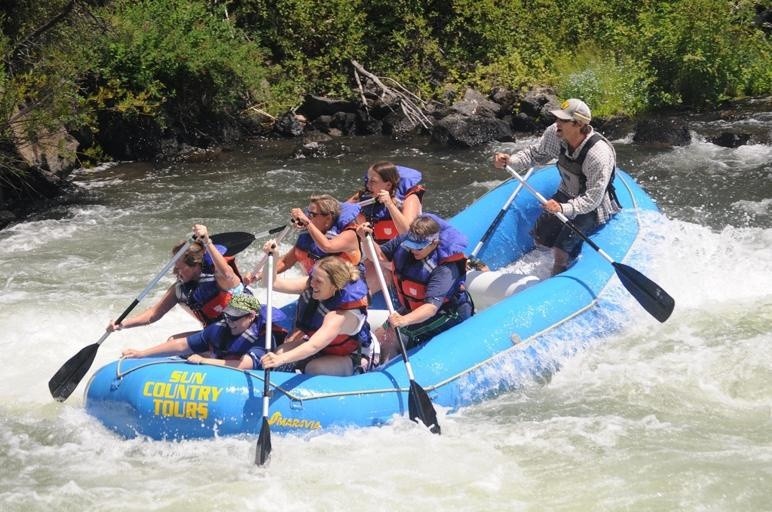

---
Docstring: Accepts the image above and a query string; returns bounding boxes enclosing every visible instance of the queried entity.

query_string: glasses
[307,211,329,218]
[226,314,250,321]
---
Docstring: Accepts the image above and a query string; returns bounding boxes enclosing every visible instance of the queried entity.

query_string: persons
[106,223,245,332]
[242,194,367,286]
[494,98,622,277]
[122,293,278,370]
[344,161,426,295]
[259,238,381,376]
[356,216,473,365]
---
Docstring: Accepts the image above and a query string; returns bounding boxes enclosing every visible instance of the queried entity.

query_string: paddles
[366,223,441,435]
[257,244,274,465]
[205,220,304,256]
[49,234,204,402]
[502,162,675,323]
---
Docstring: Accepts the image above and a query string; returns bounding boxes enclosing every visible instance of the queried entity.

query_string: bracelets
[117,322,123,330]
[197,357,203,365]
[203,238,212,248]
[304,220,312,227]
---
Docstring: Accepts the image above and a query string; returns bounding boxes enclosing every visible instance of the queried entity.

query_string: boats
[79,154,676,449]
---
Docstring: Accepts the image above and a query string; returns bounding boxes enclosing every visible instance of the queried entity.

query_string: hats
[401,231,439,249]
[223,294,261,317]
[550,98,591,123]
